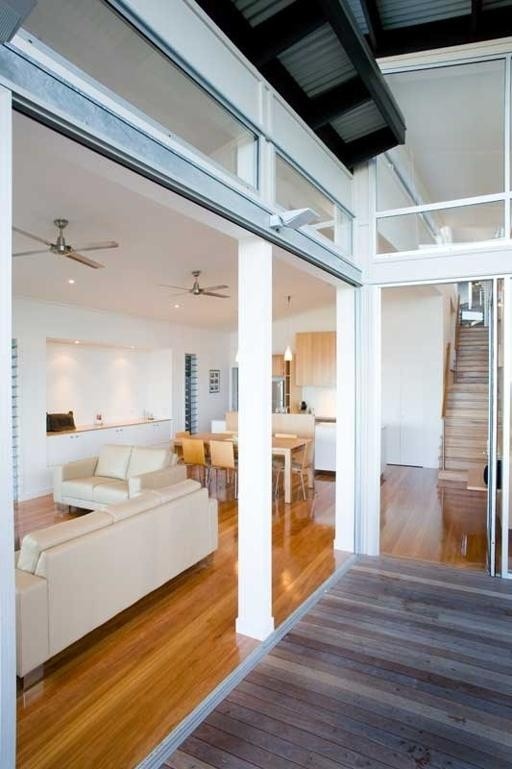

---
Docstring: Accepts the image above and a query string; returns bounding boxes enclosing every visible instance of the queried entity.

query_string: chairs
[172,431,314,505]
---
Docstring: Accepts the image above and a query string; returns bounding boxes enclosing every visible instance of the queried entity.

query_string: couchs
[53,445,188,517]
[16,478,219,694]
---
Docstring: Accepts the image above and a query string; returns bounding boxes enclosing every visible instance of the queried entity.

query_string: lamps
[283,296,293,364]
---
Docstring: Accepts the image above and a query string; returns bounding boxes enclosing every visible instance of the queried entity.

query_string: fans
[159,266,232,303]
[10,215,119,272]
